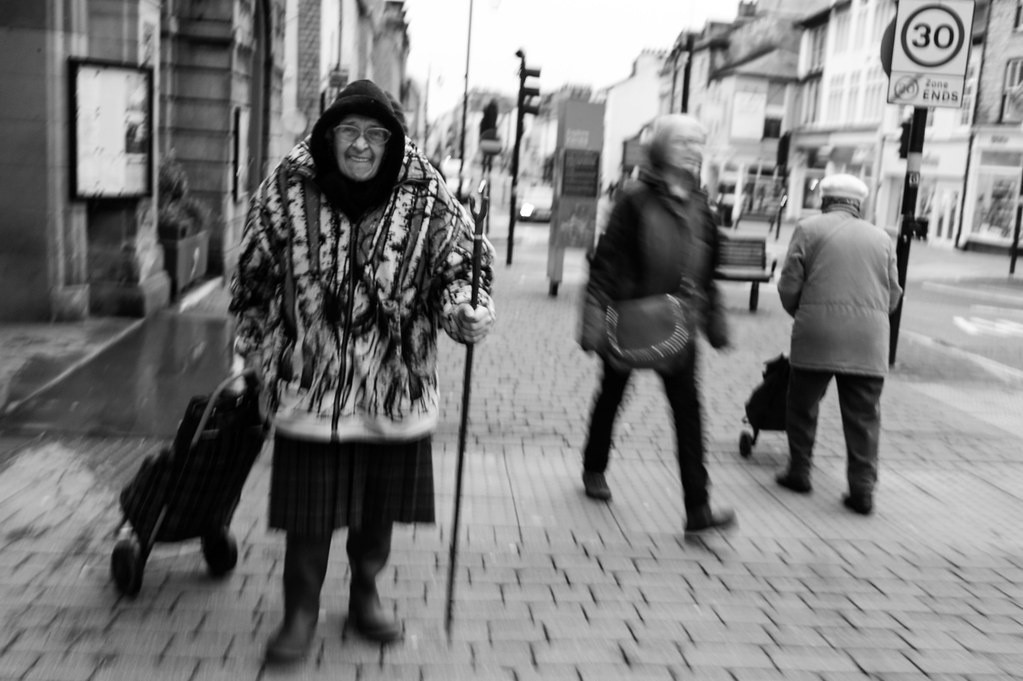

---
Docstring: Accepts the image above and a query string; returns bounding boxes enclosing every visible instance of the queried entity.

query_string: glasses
[333,124,392,146]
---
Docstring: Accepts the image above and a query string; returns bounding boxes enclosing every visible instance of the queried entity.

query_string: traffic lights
[518,65,542,117]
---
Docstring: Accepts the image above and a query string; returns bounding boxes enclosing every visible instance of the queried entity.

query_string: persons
[225,78,496,663]
[572,110,739,532]
[771,168,904,518]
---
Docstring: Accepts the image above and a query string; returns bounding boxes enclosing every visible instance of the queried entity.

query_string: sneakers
[680,501,736,540]
[771,469,813,498]
[582,469,611,501]
[841,490,877,516]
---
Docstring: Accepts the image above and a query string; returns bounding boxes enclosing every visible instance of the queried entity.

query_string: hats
[818,172,870,203]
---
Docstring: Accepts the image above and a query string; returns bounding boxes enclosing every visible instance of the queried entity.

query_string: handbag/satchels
[600,290,692,370]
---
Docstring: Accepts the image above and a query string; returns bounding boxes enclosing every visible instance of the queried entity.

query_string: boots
[343,519,404,640]
[265,528,333,663]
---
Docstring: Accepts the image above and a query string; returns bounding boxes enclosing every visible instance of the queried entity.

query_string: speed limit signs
[897,0,974,76]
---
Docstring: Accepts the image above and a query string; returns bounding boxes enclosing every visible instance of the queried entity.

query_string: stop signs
[479,130,505,156]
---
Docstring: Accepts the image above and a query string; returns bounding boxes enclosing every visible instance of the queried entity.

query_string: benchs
[584,232,768,312]
